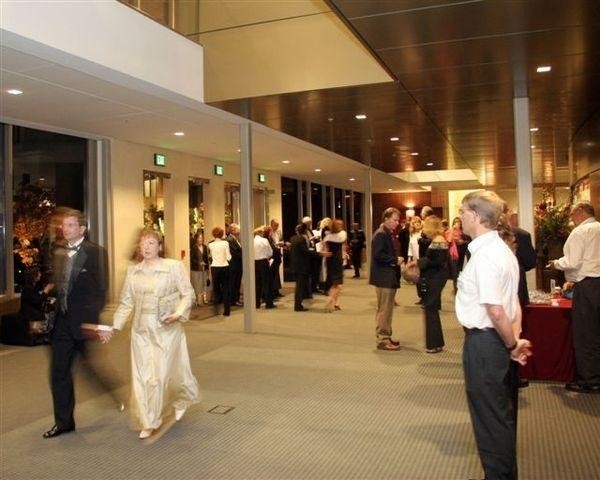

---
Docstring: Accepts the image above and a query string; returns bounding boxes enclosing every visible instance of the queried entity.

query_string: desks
[517,289,578,387]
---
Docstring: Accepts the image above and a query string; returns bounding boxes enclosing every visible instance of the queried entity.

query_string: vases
[542,235,566,259]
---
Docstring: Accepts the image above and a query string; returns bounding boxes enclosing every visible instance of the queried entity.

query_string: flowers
[530,179,580,259]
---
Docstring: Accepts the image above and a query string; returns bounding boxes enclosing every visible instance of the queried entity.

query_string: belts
[463,326,493,334]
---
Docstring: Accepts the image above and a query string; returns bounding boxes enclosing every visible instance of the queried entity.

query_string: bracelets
[508,342,520,351]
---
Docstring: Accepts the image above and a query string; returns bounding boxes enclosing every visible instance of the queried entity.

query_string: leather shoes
[139,429,153,439]
[43,424,75,438]
[174,405,186,421]
[565,381,600,394]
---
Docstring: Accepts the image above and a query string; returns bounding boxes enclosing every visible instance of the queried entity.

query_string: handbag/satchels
[207,278,211,287]
[417,275,433,298]
[158,291,181,323]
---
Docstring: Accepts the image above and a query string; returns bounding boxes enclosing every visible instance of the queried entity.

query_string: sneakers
[378,339,400,350]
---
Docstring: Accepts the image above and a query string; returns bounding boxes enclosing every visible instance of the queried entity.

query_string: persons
[495,205,536,389]
[454,189,537,480]
[100,228,196,439]
[368,206,470,353]
[40,208,125,438]
[19,267,58,345]
[288,216,347,313]
[543,201,600,393]
[253,219,284,308]
[348,223,365,278]
[191,222,243,316]
[497,213,518,258]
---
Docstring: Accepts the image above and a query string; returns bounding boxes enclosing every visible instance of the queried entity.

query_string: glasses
[458,206,475,215]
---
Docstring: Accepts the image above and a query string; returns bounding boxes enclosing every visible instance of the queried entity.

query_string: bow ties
[64,245,79,251]
